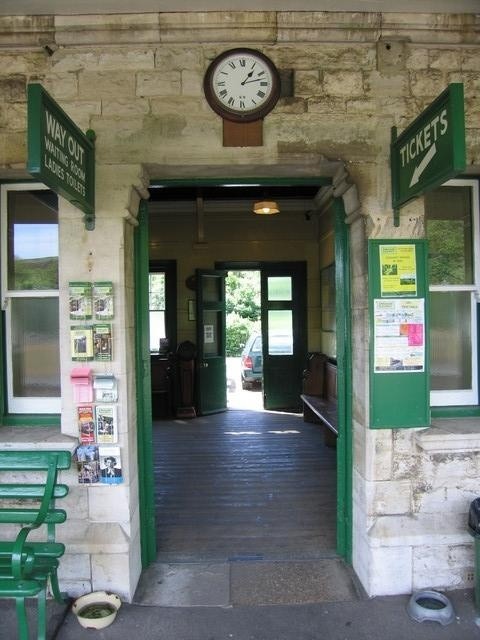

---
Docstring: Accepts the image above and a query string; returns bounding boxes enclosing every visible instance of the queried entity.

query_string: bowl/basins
[71,591,122,630]
[407,591,456,624]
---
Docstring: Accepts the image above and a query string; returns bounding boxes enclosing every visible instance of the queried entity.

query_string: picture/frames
[188,300,215,343]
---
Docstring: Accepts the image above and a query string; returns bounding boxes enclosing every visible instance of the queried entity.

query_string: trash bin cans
[466,498,480,607]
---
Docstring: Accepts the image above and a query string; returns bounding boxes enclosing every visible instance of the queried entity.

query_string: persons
[101,457,121,477]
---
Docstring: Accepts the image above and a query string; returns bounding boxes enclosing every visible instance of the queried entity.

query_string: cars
[240,332,292,390]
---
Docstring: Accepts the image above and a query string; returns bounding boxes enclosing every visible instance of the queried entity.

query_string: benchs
[301,353,339,447]
[0,452,71,640]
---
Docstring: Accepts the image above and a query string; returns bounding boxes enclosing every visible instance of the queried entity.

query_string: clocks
[203,48,281,121]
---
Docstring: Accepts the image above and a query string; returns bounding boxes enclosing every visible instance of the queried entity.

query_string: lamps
[254,201,279,216]
